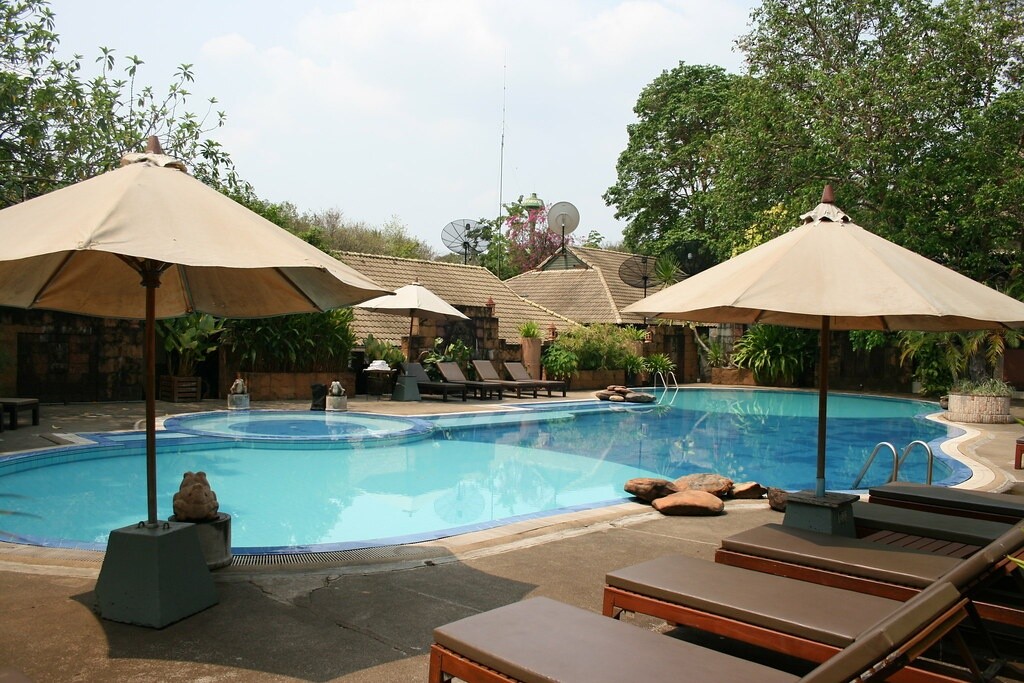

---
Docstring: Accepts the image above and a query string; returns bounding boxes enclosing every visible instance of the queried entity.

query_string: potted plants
[626,357,652,387]
[707,337,725,385]
[156,314,227,403]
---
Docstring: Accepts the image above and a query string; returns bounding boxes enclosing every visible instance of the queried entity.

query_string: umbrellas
[620,185,1024,496]
[351,276,471,362]
[0,135,397,521]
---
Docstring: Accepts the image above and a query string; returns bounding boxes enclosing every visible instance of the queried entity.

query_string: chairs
[504,362,567,397]
[400,362,467,402]
[472,360,537,399]
[436,362,502,400]
[429,480,1024,683]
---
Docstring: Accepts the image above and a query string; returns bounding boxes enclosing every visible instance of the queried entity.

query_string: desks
[363,370,398,401]
[0,398,41,428]
